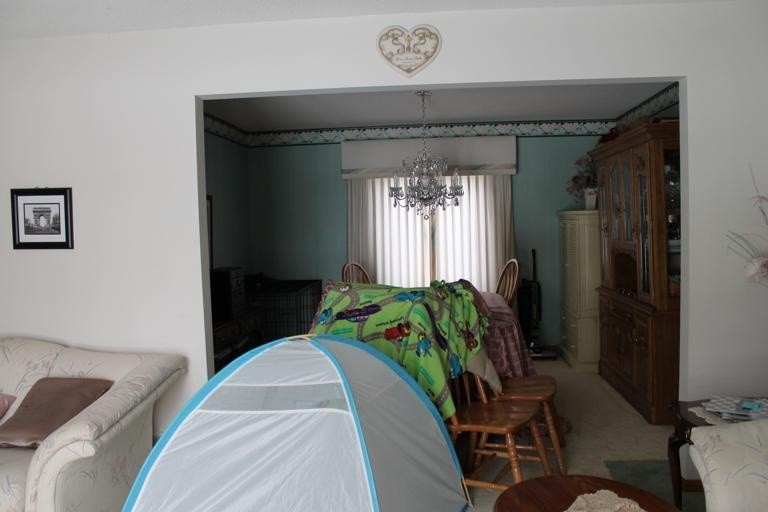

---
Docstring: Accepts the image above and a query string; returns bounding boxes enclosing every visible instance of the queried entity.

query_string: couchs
[0,337,188,512]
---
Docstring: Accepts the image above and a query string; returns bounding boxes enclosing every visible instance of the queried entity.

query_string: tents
[126,335,473,512]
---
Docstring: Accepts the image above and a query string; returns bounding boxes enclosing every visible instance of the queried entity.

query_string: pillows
[0,377,113,450]
[0,395,17,420]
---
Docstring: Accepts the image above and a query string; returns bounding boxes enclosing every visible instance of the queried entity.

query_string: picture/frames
[11,190,73,250]
[206,194,214,272]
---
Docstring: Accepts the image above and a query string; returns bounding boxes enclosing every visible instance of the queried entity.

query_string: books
[705,392,766,421]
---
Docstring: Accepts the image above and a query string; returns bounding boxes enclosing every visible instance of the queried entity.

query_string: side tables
[669,397,767,510]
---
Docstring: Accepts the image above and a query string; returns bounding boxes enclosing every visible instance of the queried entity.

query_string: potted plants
[566,153,601,209]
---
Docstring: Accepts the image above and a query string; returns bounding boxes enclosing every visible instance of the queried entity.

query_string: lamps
[387,89,463,219]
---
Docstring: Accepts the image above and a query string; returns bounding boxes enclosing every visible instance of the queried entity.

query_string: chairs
[343,261,371,286]
[495,257,518,302]
[466,355,567,475]
[440,375,553,492]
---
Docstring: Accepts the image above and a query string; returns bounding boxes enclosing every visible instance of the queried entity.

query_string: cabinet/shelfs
[211,266,250,362]
[557,210,602,374]
[585,119,681,425]
